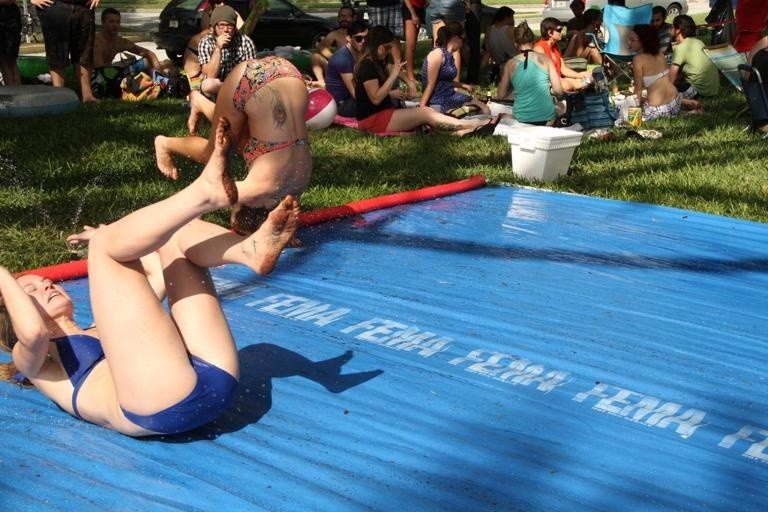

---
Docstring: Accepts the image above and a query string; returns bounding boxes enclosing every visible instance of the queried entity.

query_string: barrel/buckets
[628,107,642,130]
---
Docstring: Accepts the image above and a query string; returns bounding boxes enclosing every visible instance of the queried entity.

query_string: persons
[154,56,314,250]
[1,2,767,153]
[1,115,303,439]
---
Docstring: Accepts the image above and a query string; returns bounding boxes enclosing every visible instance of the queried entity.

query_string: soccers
[304,88,338,129]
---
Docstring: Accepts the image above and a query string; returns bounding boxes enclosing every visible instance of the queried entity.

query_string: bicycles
[20,4,44,43]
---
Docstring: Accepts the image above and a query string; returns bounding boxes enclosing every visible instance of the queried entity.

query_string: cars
[153,0,342,65]
[542,0,688,29]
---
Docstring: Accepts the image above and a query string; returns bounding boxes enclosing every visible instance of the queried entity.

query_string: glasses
[355,36,368,42]
[555,27,562,33]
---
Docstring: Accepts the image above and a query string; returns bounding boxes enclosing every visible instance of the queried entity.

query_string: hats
[211,5,237,28]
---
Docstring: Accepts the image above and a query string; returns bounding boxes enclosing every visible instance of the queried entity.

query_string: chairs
[737,64,767,139]
[701,43,750,119]
[584,32,635,88]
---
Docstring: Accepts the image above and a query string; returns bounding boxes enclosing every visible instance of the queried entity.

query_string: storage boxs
[503,126,584,185]
